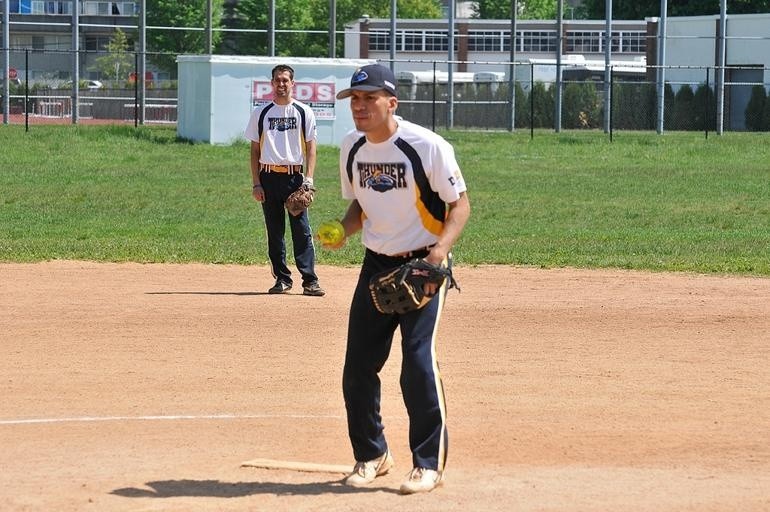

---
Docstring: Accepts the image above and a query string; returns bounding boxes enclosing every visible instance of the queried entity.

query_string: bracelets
[252,184,262,188]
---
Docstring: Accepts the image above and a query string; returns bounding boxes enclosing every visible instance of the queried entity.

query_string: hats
[336,65,398,100]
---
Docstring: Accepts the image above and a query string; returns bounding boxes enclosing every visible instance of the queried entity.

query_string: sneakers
[269,282,292,294]
[303,283,325,296]
[344,449,393,486]
[400,466,445,494]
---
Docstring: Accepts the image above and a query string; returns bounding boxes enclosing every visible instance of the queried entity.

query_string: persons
[313,64,472,493]
[244,65,326,298]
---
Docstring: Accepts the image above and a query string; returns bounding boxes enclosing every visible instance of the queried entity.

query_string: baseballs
[317,220,344,245]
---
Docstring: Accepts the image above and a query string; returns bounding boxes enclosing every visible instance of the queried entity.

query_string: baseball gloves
[286,182,315,216]
[369,257,448,314]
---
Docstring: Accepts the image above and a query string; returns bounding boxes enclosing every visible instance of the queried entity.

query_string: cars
[60,79,103,89]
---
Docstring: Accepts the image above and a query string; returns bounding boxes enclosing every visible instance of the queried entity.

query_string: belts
[379,243,437,259]
[264,164,302,174]
